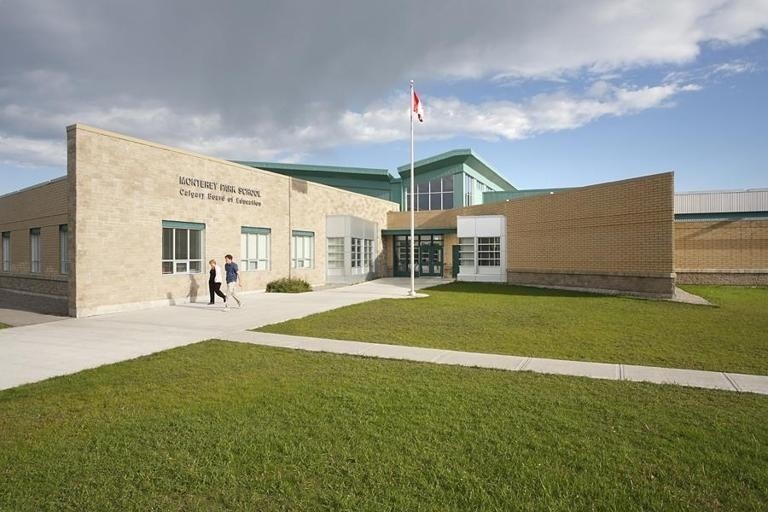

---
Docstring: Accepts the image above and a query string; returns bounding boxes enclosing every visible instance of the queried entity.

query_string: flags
[412,91,426,124]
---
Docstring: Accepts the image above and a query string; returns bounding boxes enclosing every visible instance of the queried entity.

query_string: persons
[224,253,244,312]
[207,260,225,305]
